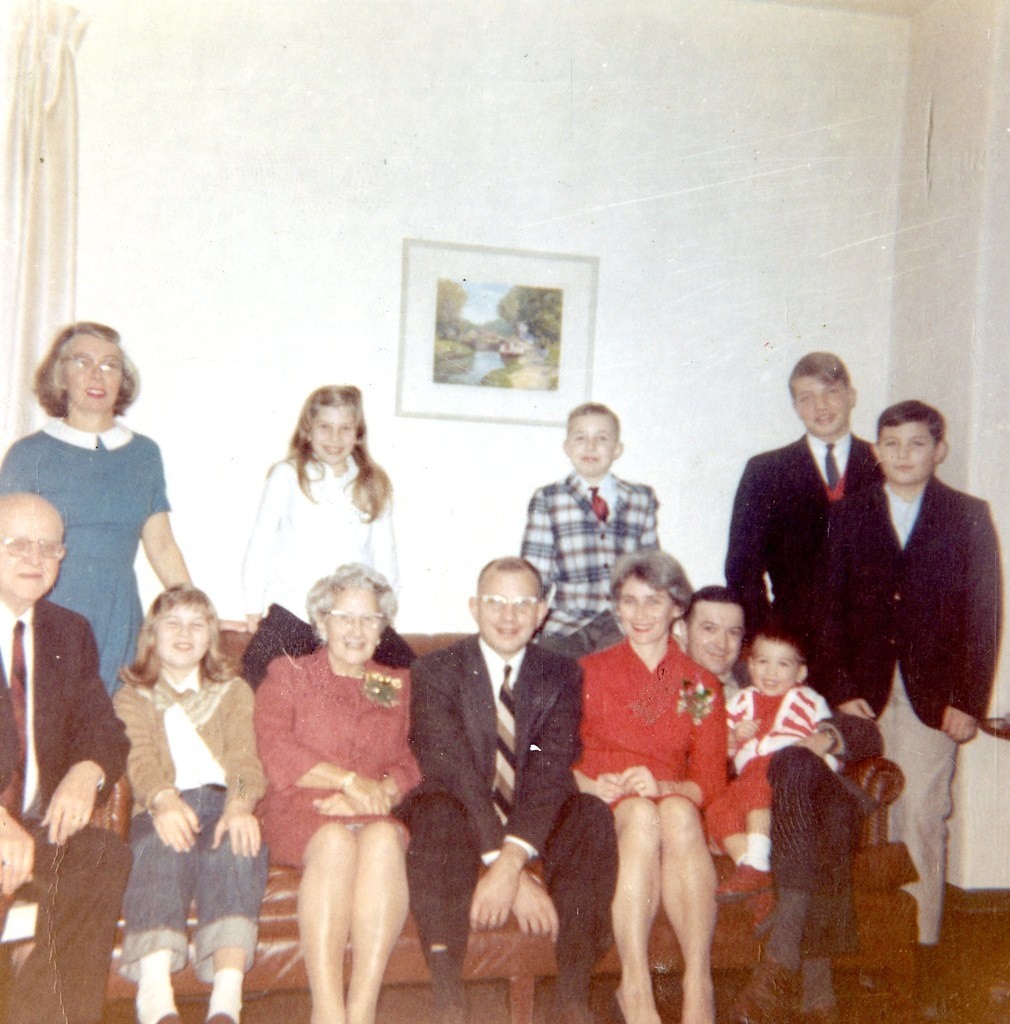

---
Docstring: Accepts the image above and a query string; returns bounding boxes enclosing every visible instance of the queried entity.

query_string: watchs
[817,728,840,756]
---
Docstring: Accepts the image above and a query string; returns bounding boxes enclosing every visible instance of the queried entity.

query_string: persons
[0,493,133,1024]
[521,404,660,655]
[0,322,195,694]
[671,585,886,1024]
[725,351,885,694]
[572,550,729,1024]
[252,565,420,1024]
[242,386,416,690]
[111,584,270,1024]
[813,402,1002,973]
[393,557,618,1024]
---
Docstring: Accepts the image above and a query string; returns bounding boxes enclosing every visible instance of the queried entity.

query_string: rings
[490,916,498,921]
[3,860,13,865]
[72,817,84,822]
[640,782,645,790]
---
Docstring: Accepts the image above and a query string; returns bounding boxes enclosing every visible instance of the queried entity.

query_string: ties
[825,443,843,490]
[0,621,26,825]
[492,665,515,829]
[589,487,609,521]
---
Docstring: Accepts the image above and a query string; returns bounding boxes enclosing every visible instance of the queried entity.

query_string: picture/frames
[399,229,598,429]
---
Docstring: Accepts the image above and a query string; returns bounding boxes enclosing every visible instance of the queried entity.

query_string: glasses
[0,537,66,561]
[321,610,384,628]
[474,595,540,611]
[61,354,122,373]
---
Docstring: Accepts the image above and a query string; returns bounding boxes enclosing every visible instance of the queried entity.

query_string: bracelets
[151,788,176,814]
[340,771,358,795]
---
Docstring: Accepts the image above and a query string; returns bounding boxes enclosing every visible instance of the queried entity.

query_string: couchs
[97,621,919,1024]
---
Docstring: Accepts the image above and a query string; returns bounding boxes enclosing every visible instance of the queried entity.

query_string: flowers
[362,671,403,711]
[676,680,715,726]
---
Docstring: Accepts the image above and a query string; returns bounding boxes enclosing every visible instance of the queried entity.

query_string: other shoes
[136,995,183,1024]
[742,891,776,939]
[715,863,774,903]
[729,961,798,1022]
[203,996,243,1023]
